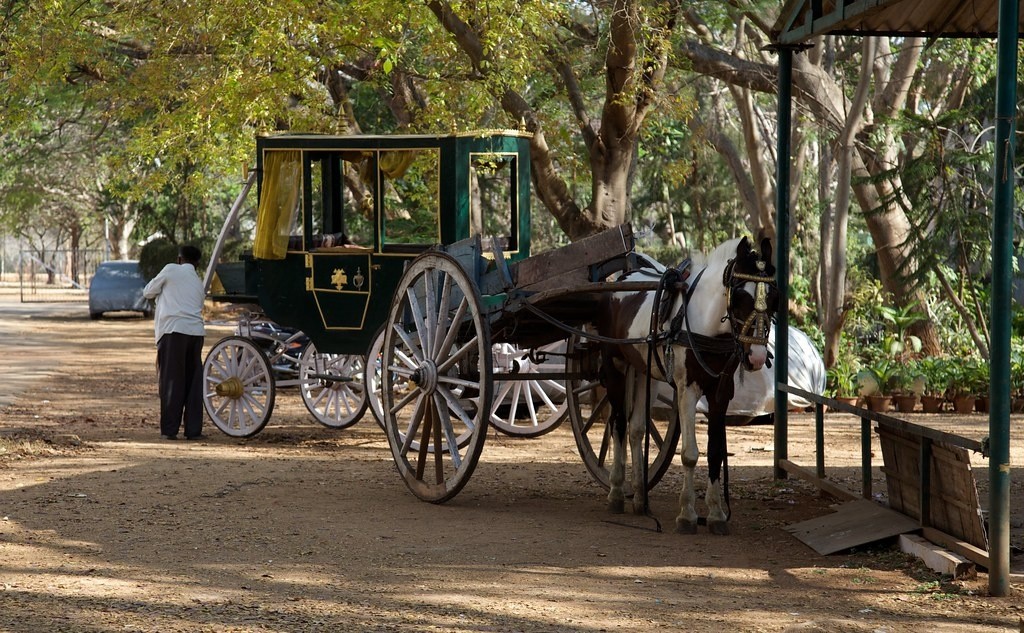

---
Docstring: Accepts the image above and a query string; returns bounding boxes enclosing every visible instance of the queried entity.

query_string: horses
[608,235,778,534]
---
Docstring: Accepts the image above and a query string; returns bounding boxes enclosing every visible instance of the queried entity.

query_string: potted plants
[811,299,1024,416]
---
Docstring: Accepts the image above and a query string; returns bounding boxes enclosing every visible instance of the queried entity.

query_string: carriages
[203,104,780,537]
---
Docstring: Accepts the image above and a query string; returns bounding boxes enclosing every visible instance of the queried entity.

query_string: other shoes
[188,434,206,440]
[167,434,177,439]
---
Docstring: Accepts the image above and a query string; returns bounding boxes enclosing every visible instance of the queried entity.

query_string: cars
[89,261,153,320]
[437,251,826,426]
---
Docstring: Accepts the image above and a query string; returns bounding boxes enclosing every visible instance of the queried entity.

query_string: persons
[142,245,208,440]
